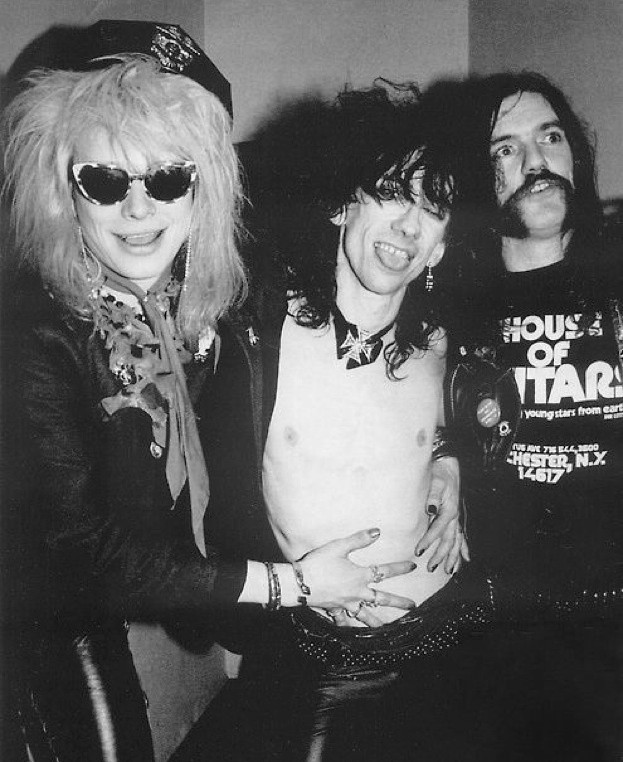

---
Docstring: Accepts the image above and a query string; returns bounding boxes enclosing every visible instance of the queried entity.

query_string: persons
[0,15,623,762]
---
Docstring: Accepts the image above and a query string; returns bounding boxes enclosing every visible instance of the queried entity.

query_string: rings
[328,610,344,617]
[369,566,384,583]
[347,601,365,618]
[364,588,380,607]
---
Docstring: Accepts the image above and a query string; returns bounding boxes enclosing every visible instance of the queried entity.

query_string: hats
[65,18,233,121]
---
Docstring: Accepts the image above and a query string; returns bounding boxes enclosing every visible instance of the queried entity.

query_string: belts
[283,598,489,673]
[504,586,623,620]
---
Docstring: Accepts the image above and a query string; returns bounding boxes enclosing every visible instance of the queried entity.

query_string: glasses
[70,161,199,206]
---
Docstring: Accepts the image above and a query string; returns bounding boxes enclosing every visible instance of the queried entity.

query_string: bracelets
[262,561,283,613]
[290,561,311,609]
